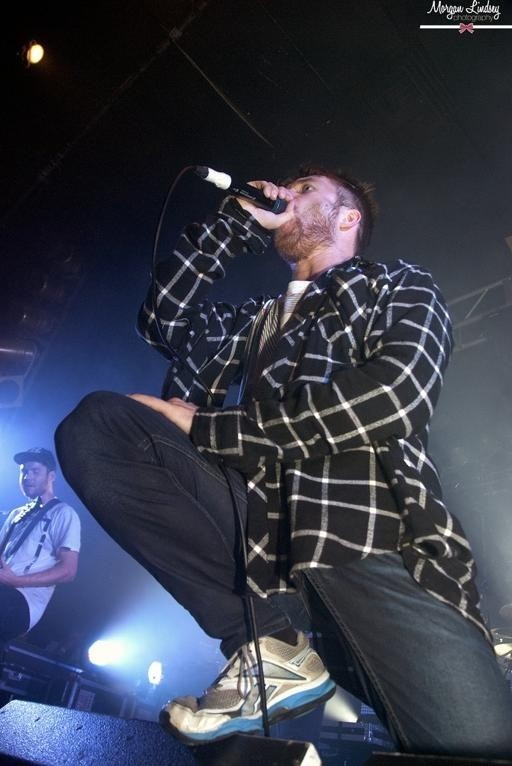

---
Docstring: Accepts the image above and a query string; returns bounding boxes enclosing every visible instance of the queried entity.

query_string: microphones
[194,165,290,215]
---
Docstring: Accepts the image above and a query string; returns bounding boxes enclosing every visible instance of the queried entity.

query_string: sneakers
[156,624,336,747]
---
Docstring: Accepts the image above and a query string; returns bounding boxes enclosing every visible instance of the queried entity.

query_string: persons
[52,162,512,761]
[0,445,83,648]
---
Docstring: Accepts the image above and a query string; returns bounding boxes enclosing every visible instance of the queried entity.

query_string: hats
[12,447,57,470]
